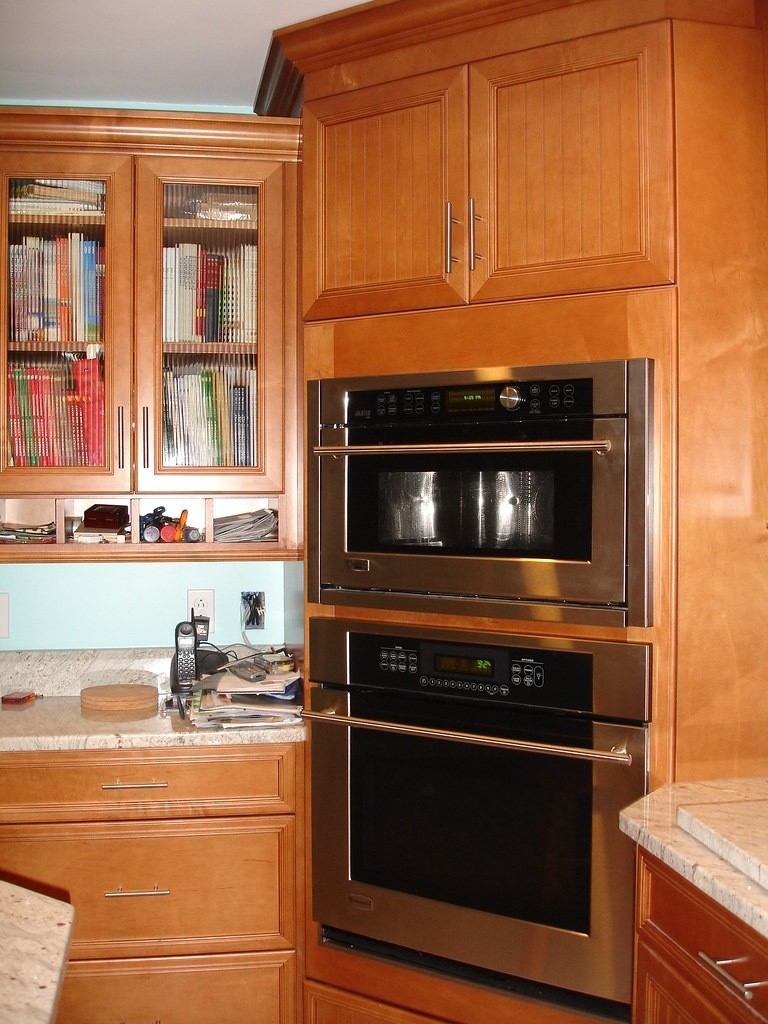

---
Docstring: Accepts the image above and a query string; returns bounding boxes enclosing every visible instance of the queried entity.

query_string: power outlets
[187,589,216,635]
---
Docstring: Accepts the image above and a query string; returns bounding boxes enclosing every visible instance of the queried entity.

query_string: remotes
[229,660,266,682]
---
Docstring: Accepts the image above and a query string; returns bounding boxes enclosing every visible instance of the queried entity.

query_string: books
[162,184,259,220]
[184,651,306,729]
[8,232,106,343]
[8,352,106,467]
[0,522,57,544]
[162,354,260,467]
[164,243,259,343]
[9,180,106,216]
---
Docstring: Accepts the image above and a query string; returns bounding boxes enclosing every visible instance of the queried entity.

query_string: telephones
[171,607,229,694]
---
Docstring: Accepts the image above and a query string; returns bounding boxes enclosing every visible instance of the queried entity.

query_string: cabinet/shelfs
[628,777,768,1024]
[0,696,308,1024]
[0,102,302,560]
[253,0,768,362]
